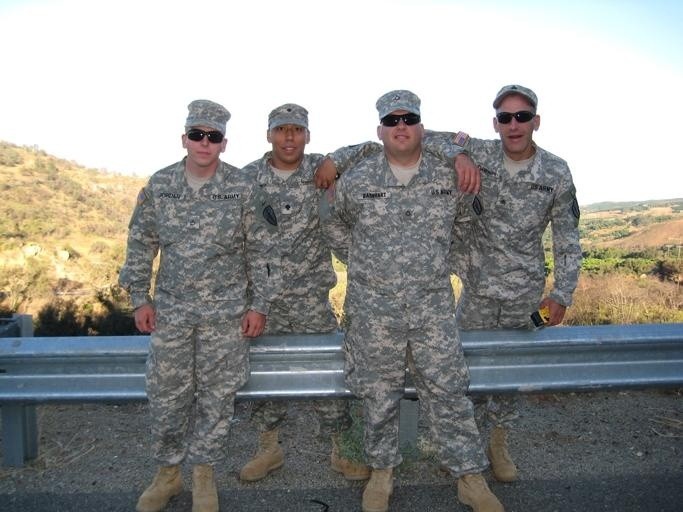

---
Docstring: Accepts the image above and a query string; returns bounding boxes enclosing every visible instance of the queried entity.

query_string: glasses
[498,111,533,124]
[382,114,419,126]
[186,128,223,143]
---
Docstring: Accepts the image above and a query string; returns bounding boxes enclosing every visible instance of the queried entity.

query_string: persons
[316,88,511,512]
[233,102,383,481]
[116,97,273,511]
[420,83,581,482]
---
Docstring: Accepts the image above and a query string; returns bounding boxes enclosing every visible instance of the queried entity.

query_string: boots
[329,433,370,481]
[137,467,185,511]
[240,431,283,480]
[490,428,517,483]
[193,465,219,512]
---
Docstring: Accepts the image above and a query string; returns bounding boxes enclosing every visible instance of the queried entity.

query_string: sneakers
[363,469,394,512]
[457,474,504,511]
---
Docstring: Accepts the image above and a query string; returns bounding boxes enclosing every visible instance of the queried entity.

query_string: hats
[183,100,230,133]
[267,104,308,132]
[494,85,538,107]
[377,90,421,123]
